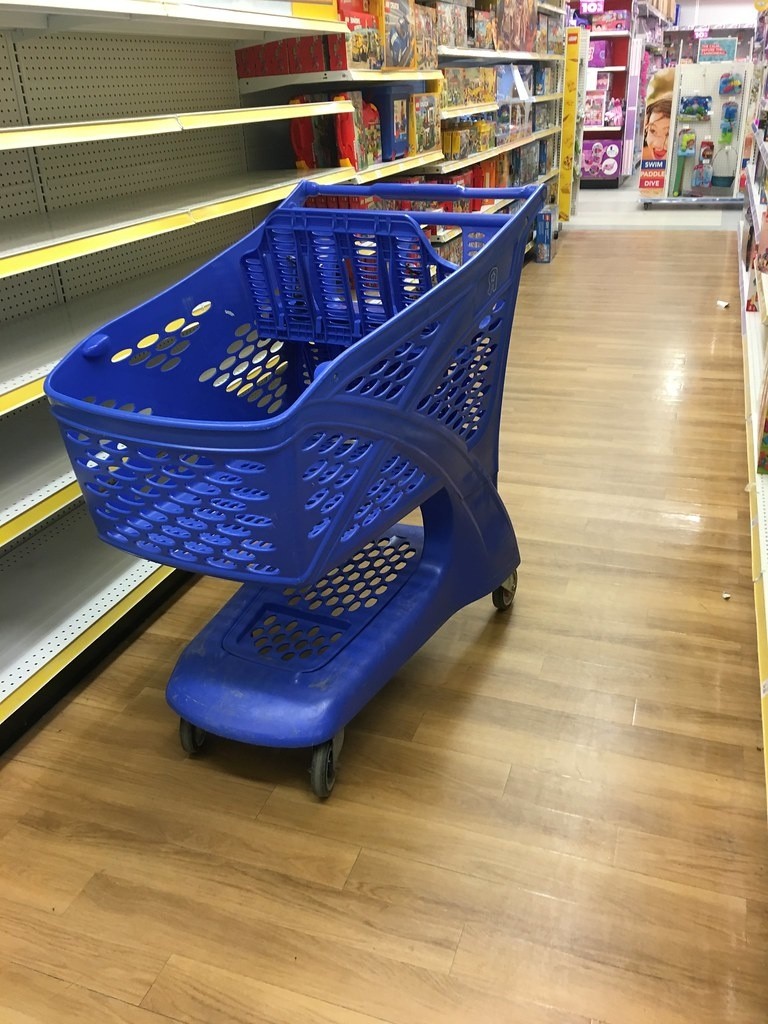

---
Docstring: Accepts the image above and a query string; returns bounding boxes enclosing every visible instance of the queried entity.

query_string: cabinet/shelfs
[664,24,754,65]
[235,50,443,320]
[568,0,672,185]
[0,2,361,759]
[438,46,564,277]
[738,2,768,785]
[641,59,752,210]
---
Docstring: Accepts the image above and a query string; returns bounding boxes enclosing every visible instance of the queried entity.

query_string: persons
[640,97,672,174]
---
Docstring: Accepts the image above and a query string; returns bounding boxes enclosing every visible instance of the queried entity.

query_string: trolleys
[45,184,556,797]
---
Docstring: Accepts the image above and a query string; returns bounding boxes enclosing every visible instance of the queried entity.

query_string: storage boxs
[237,0,673,286]
